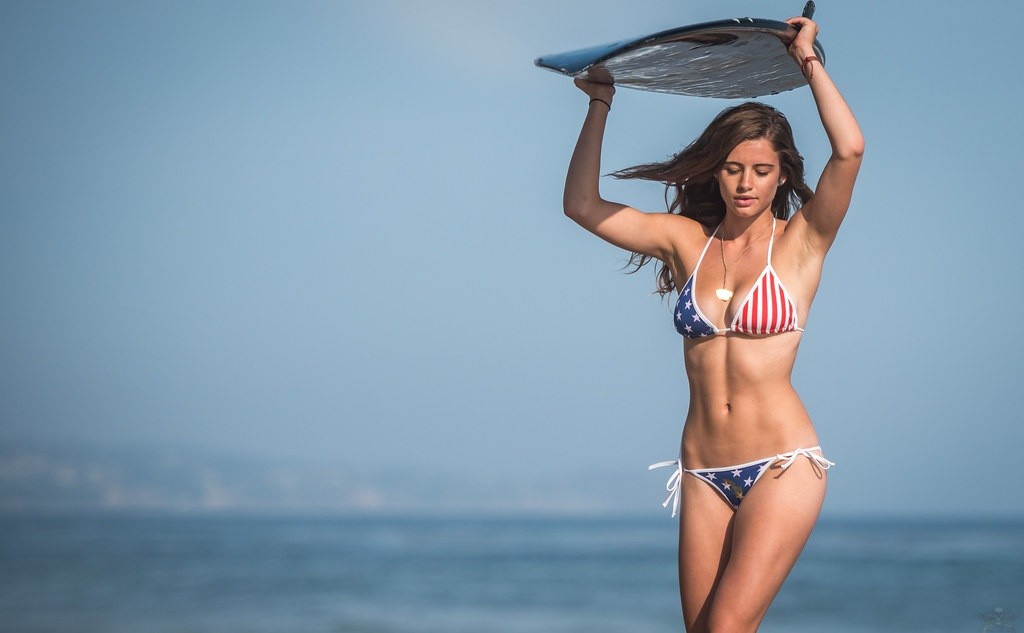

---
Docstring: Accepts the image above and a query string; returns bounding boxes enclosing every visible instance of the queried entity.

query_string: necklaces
[715,213,774,300]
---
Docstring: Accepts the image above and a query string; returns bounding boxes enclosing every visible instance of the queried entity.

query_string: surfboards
[533,16,827,100]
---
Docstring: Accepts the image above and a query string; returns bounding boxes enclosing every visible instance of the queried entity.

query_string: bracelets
[801,56,822,78]
[589,98,611,111]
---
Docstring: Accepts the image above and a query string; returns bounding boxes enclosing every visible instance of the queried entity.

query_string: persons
[562,16,864,632]
